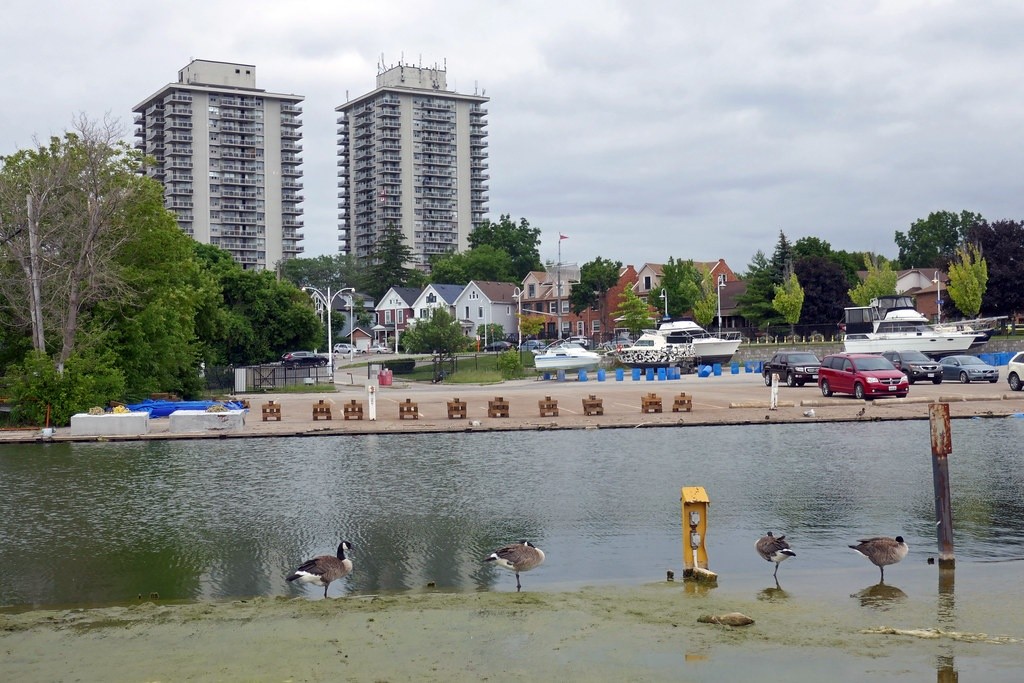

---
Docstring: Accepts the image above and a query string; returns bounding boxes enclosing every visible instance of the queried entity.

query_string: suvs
[333,343,360,354]
[281,351,329,370]
[566,335,591,349]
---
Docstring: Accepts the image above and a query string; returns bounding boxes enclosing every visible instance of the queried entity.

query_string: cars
[522,340,547,351]
[818,353,909,400]
[602,337,632,350]
[938,355,999,383]
[882,350,944,385]
[1007,351,1024,391]
[361,345,393,354]
[506,333,527,344]
[762,351,822,387]
[486,341,515,351]
[998,324,1024,335]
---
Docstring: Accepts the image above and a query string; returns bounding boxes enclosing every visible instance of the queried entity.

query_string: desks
[169,408,249,433]
[70,411,150,435]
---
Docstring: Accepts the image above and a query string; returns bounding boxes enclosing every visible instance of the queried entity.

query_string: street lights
[481,299,488,353]
[932,271,941,324]
[511,287,522,350]
[659,289,670,321]
[717,274,728,338]
[304,285,356,384]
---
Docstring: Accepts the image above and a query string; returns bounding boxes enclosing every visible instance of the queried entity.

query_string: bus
[614,328,658,338]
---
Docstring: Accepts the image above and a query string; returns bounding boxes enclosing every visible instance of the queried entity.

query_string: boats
[839,294,1009,356]
[535,344,602,372]
[604,319,741,372]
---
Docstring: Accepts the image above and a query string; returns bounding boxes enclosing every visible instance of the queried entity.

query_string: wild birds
[285,540,357,598]
[755,531,796,575]
[847,536,909,577]
[856,407,866,417]
[483,539,546,587]
